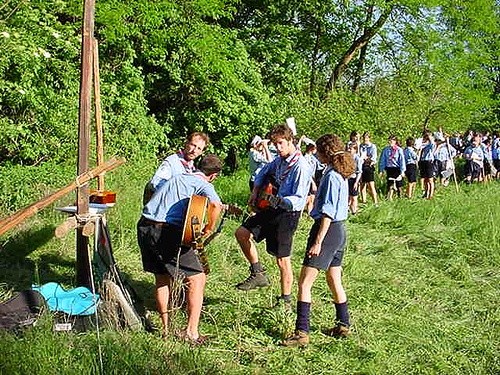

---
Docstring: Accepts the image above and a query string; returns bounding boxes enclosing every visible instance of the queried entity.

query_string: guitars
[249,180,282,214]
[180,196,244,249]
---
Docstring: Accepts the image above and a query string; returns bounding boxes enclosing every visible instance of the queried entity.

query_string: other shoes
[178,330,209,345]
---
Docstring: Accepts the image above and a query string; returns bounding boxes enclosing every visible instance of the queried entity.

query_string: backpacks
[99,279,142,332]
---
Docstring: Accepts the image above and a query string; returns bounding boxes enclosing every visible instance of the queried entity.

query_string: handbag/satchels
[1,289,50,331]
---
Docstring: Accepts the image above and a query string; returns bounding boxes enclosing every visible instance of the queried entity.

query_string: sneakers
[324,320,348,340]
[262,301,292,316]
[279,330,309,347]
[238,268,267,290]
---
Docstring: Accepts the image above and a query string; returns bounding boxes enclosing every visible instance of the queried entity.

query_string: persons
[235,126,311,308]
[280,134,355,347]
[137,132,222,345]
[243,130,500,216]
